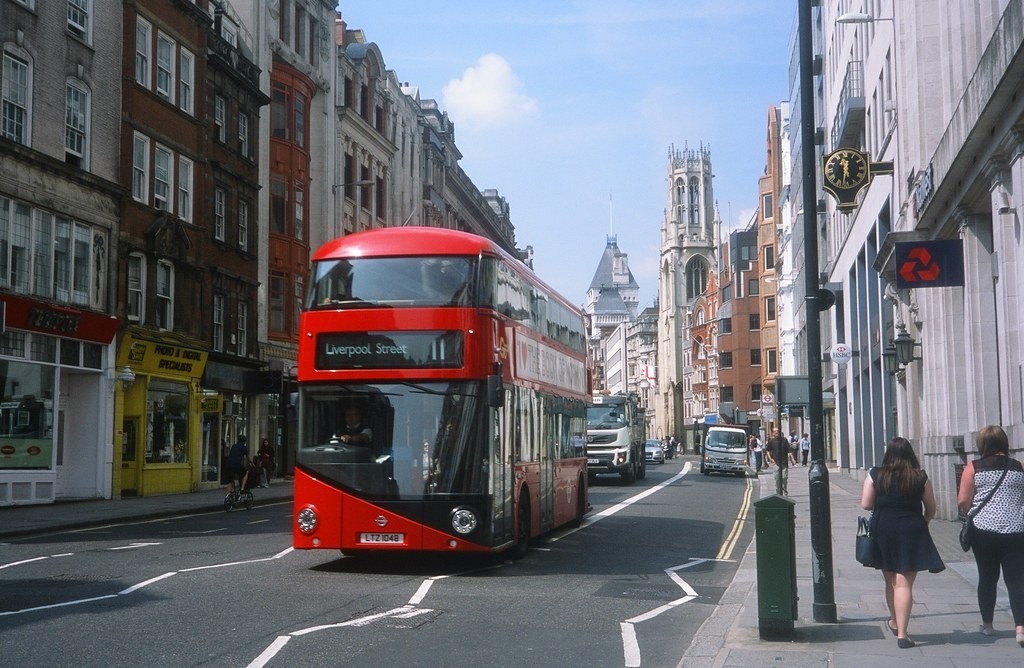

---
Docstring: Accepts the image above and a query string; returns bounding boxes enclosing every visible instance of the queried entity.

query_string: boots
[978,622,995,636]
[1016,626,1024,642]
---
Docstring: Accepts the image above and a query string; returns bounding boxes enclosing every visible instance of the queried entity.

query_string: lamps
[836,13,894,24]
[893,324,923,366]
[105,364,135,397]
[880,339,900,377]
[332,180,375,193]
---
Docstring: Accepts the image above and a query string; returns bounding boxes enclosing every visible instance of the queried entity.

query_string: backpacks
[752,439,757,448]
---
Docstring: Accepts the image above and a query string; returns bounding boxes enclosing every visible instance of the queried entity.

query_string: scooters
[662,443,673,459]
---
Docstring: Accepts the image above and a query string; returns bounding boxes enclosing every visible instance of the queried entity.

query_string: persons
[957,425,1024,647]
[657,435,685,455]
[861,437,946,649]
[695,443,700,454]
[227,435,252,494]
[749,428,810,495]
[335,409,373,445]
[257,439,275,487]
[417,440,441,493]
[443,420,454,439]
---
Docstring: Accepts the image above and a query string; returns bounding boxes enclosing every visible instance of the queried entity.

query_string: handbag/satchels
[855,516,876,565]
[240,446,249,469]
[960,517,974,552]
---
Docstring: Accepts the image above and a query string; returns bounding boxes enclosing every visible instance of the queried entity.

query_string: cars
[645,439,664,464]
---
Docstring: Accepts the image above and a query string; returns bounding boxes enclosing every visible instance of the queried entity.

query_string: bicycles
[224,467,260,512]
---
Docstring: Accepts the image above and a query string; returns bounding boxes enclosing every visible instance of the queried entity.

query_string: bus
[0,395,52,467]
[287,227,594,560]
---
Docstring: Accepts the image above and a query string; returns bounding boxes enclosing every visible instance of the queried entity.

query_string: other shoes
[898,638,915,648]
[888,618,898,636]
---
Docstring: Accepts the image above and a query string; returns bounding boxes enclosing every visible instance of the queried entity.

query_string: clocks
[822,147,871,216]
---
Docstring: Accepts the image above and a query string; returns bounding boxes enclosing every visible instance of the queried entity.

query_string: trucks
[695,424,757,476]
[586,390,646,484]
